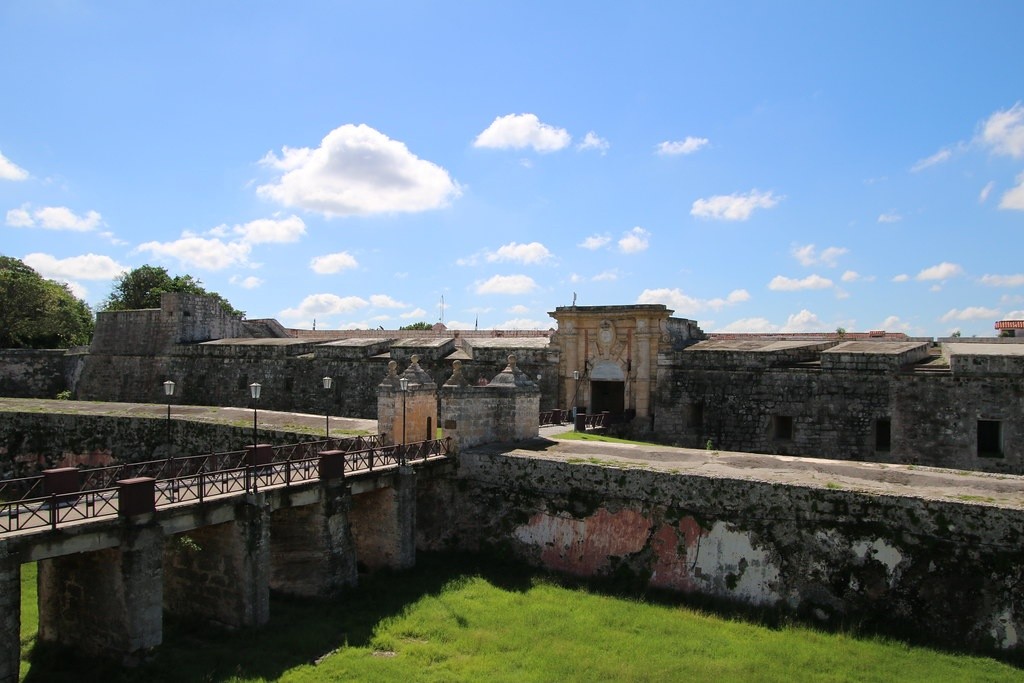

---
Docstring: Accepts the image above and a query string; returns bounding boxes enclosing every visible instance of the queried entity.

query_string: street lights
[249,382,262,494]
[399,377,409,466]
[163,380,177,500]
[573,371,580,414]
[323,376,333,451]
